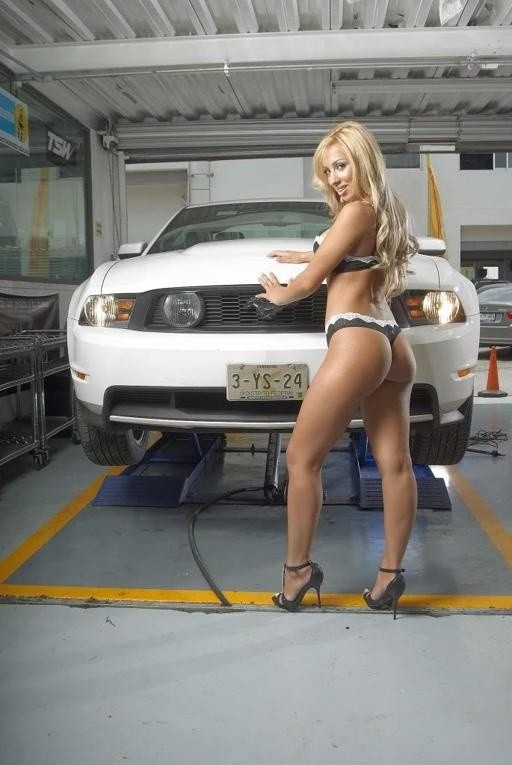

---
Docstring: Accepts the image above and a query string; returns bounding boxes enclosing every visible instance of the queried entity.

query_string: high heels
[358,565,409,621]
[271,560,326,613]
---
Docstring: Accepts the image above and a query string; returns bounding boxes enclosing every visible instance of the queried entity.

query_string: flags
[30,162,50,277]
[427,155,449,260]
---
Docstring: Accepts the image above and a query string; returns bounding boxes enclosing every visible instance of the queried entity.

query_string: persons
[253,121,418,619]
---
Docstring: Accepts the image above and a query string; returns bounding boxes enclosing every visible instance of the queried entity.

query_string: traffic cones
[478,344,508,399]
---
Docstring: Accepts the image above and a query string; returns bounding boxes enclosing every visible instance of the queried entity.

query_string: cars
[64,191,486,472]
[473,281,511,357]
